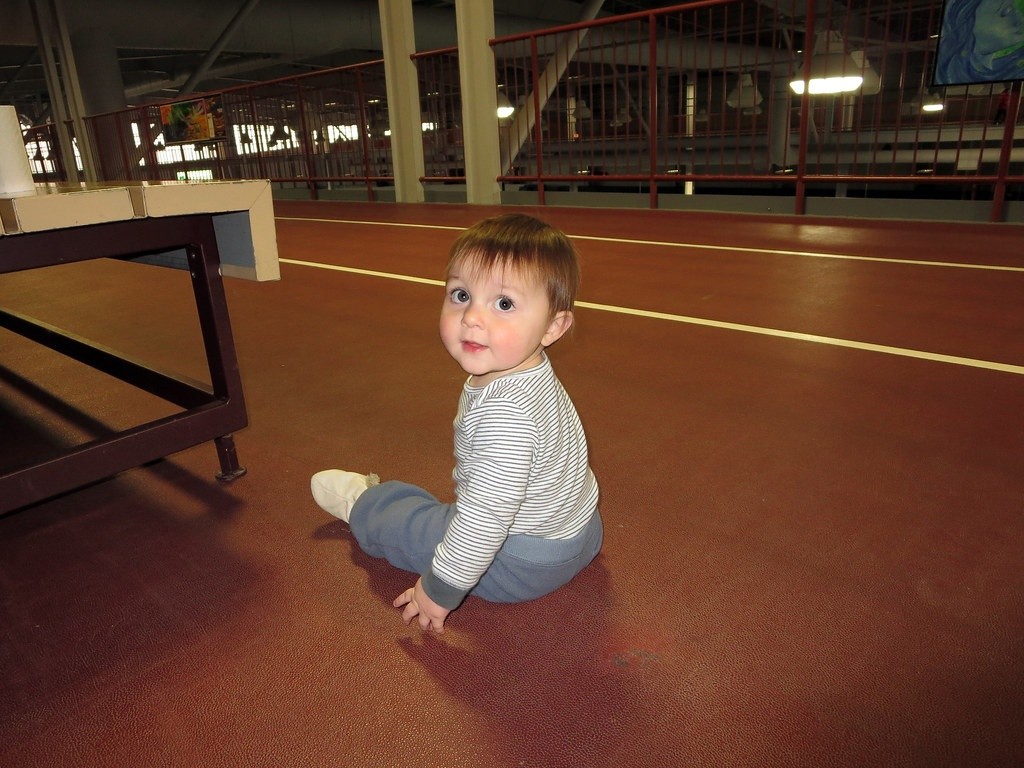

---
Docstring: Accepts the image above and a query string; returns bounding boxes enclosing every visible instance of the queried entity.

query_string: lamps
[26,0,1024,163]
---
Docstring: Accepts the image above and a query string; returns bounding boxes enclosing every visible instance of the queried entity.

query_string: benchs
[0,102,281,517]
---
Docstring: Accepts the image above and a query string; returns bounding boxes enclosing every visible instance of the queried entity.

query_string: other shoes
[311,468,379,523]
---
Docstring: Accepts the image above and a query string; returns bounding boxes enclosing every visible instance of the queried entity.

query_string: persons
[310,215,605,634]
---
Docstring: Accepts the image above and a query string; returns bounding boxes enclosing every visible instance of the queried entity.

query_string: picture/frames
[159,98,211,145]
[158,92,227,146]
[930,1,1024,88]
